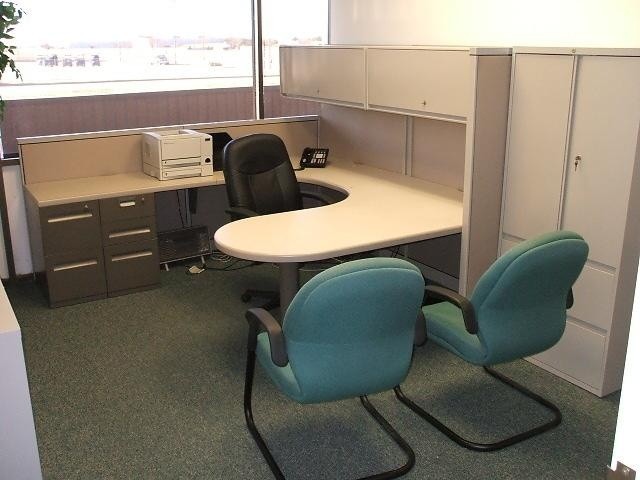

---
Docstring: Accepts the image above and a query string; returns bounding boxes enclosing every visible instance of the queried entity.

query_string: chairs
[240,251,435,480]
[220,131,339,313]
[392,224,593,455]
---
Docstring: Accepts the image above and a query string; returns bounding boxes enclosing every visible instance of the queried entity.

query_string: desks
[23,152,470,331]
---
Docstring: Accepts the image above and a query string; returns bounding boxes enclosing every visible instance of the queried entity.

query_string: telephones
[300,147,329,168]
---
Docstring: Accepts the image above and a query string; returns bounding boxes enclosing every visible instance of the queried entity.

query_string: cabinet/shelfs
[21,185,108,311]
[493,45,640,398]
[94,187,165,298]
[275,40,516,302]
